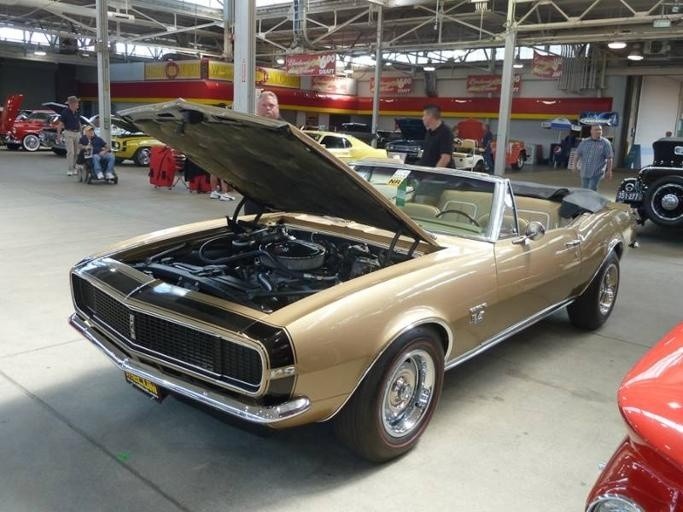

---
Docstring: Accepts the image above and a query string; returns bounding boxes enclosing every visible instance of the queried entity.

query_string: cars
[584,315,682,511]
[615,131,682,231]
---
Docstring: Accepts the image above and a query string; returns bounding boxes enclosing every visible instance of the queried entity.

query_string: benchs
[436,191,562,239]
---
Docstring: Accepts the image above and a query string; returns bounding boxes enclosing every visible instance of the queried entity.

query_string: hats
[63,96,81,103]
[85,126,94,132]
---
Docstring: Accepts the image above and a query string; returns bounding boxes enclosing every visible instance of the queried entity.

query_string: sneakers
[97,171,105,180]
[210,191,221,199]
[106,172,115,180]
[66,169,79,176]
[219,193,236,201]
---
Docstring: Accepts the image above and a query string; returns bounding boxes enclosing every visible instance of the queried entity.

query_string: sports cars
[67,99,640,462]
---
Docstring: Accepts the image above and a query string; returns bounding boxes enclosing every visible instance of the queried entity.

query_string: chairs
[398,203,439,219]
[474,215,531,235]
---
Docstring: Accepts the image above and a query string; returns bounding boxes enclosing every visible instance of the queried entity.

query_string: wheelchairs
[73,138,118,185]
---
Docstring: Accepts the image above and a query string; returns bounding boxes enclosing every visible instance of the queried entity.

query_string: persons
[244,91,280,215]
[417,105,455,182]
[572,123,613,192]
[54,95,86,176]
[78,124,115,181]
[479,123,492,173]
[208,174,234,203]
